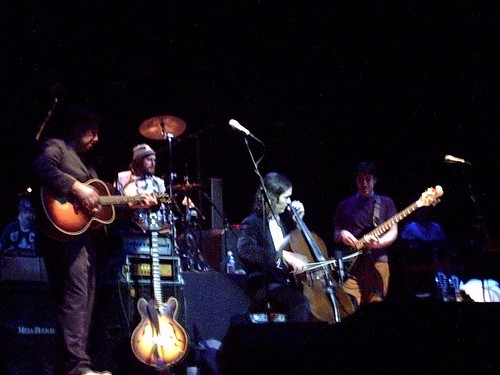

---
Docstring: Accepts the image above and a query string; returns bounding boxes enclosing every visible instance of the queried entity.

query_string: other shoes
[82,369,112,375]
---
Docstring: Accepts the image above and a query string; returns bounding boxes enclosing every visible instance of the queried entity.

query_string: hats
[133,144,156,161]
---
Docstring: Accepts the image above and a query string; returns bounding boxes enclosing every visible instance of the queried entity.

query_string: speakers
[177,269,249,349]
[1,279,70,360]
[205,177,224,230]
[216,298,500,375]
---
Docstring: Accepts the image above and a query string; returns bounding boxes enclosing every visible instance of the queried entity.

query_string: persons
[396,194,449,308]
[29,104,159,375]
[235,173,310,324]
[113,143,167,273]
[334,159,399,301]
[0,197,43,289]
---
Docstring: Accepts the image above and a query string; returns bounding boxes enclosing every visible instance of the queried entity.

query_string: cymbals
[173,184,202,193]
[138,115,187,141]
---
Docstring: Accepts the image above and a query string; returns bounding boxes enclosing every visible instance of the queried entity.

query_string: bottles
[435,268,463,302]
[227,250,235,274]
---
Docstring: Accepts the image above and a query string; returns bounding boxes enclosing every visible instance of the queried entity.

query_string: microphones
[444,154,465,165]
[229,119,265,145]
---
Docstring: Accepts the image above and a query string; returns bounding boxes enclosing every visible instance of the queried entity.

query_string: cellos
[279,201,362,324]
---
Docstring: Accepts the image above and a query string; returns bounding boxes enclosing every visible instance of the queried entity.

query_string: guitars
[329,184,444,273]
[129,207,192,375]
[34,178,172,242]
